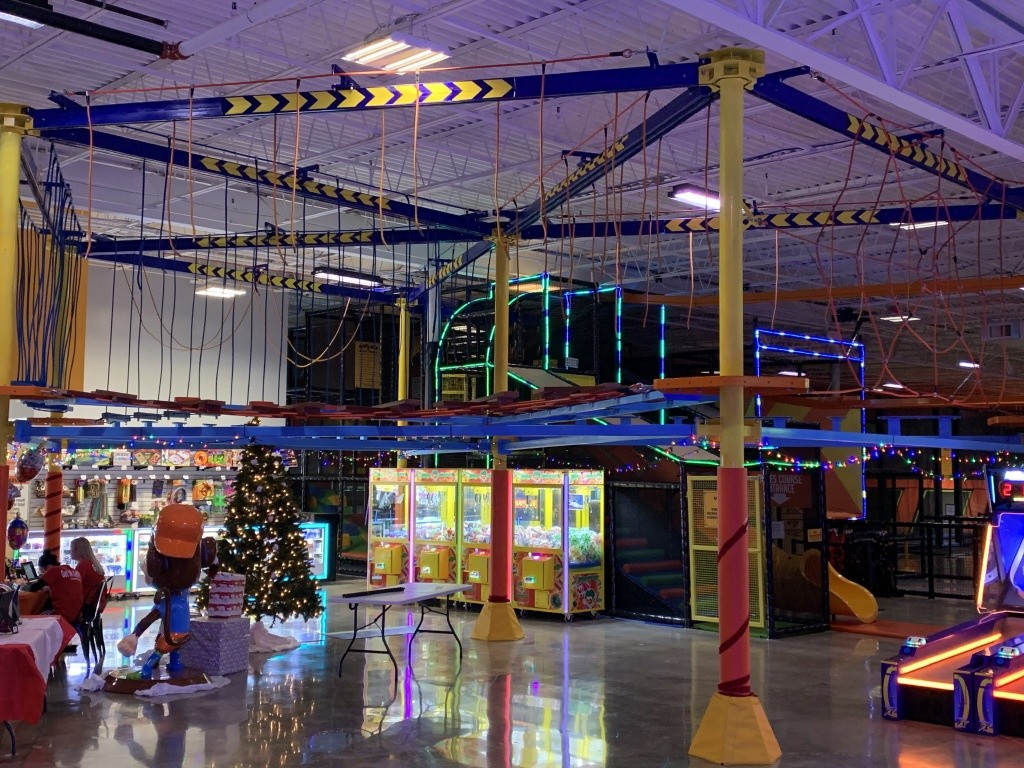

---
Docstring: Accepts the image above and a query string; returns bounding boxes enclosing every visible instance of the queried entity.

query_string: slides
[560,418,879,629]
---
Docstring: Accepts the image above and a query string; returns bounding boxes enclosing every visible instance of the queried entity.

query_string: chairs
[78,575,115,659]
[66,579,106,670]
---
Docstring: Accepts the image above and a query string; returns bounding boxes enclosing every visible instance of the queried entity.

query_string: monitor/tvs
[999,481,1024,498]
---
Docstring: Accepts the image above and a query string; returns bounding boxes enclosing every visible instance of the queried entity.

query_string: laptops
[22,562,37,581]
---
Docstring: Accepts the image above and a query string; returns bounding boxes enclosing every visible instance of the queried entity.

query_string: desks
[18,586,49,616]
[0,615,77,757]
[325,581,473,682]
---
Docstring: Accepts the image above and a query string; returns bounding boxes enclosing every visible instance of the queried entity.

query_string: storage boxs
[208,571,246,618]
[177,616,251,677]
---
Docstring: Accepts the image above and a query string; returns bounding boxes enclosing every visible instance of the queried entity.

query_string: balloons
[6,437,46,551]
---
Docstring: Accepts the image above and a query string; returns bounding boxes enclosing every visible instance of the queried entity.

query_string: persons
[70,537,108,615]
[20,553,82,620]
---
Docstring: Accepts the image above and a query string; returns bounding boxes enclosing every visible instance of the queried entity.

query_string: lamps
[668,181,721,212]
[340,32,453,76]
